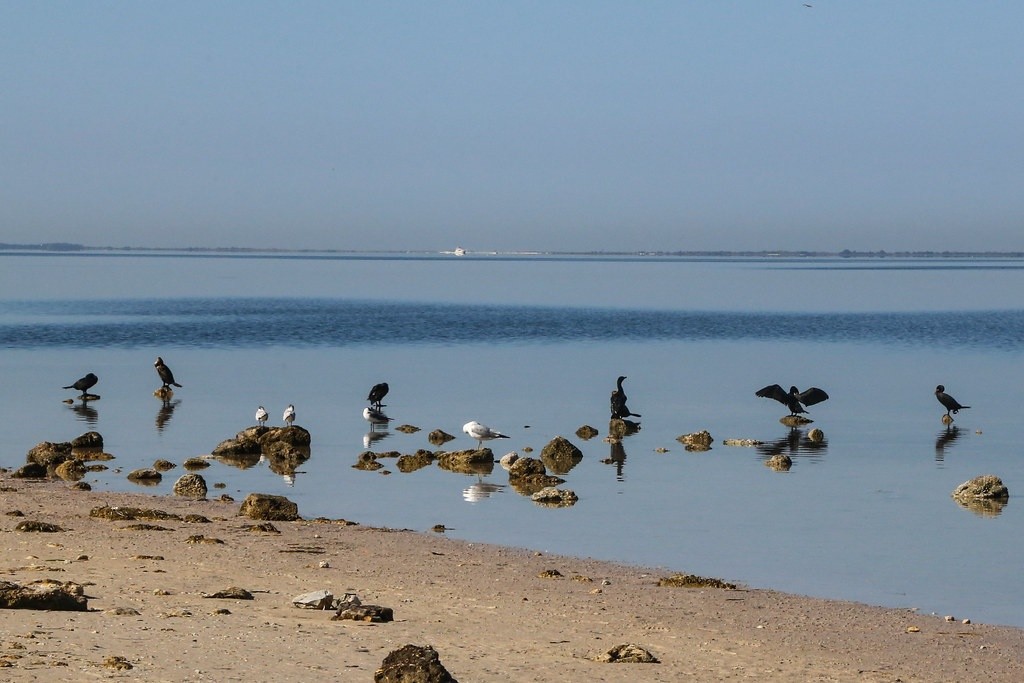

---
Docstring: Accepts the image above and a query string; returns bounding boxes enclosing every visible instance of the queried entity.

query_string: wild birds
[363,431,389,448]
[362,382,389,433]
[153,356,183,387]
[934,386,974,417]
[61,372,98,408]
[463,420,511,450]
[757,382,830,417]
[282,404,296,427]
[611,375,644,423]
[462,474,510,503]
[256,406,268,426]
